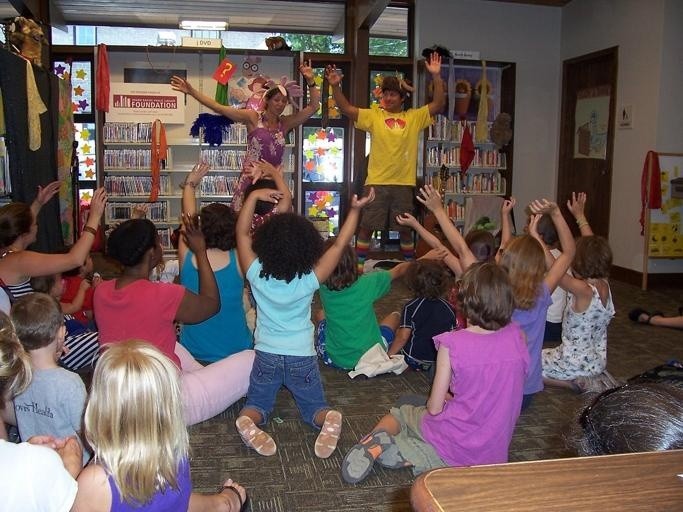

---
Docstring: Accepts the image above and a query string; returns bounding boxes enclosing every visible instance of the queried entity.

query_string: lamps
[178,17,232,31]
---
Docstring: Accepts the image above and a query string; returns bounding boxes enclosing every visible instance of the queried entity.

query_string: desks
[408,438,682,512]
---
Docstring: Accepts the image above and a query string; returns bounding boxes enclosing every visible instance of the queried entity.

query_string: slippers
[218,479,249,512]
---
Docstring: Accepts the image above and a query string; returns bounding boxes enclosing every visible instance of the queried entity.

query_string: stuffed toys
[11,17,50,68]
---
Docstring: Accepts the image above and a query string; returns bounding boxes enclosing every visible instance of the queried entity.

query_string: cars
[303,137,344,208]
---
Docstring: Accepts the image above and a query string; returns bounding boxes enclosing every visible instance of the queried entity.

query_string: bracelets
[307,82,316,88]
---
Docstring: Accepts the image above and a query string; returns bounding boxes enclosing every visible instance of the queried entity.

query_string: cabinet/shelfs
[420,137,511,246]
[102,137,295,262]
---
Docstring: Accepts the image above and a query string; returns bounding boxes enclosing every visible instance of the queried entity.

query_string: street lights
[156,31,177,46]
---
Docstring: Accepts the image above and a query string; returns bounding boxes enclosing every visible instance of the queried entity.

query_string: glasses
[579,382,630,444]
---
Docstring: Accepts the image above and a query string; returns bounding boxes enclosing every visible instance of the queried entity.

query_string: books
[426,113,502,236]
[196,126,295,213]
[103,122,173,250]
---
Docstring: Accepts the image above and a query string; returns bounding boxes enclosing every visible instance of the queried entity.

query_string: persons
[628,307,683,329]
[323,51,447,275]
[170,58,321,242]
[564,379,683,456]
[1,157,615,512]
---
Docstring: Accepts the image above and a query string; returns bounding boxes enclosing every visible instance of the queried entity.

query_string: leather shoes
[629,309,664,326]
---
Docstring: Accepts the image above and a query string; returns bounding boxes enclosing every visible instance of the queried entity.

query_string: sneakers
[235,415,277,456]
[342,429,393,483]
[377,442,416,470]
[314,410,343,459]
